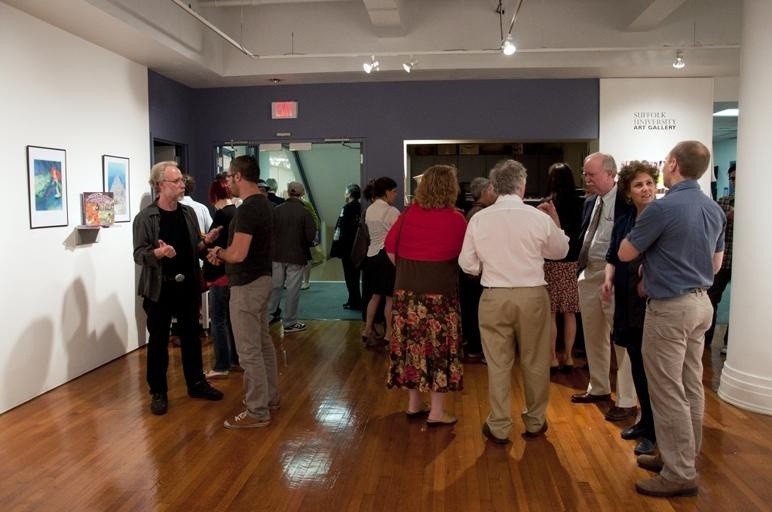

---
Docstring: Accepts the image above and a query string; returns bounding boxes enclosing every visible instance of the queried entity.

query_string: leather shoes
[343,304,363,310]
[621,423,642,439]
[572,392,611,402]
[606,407,638,420]
[634,438,656,454]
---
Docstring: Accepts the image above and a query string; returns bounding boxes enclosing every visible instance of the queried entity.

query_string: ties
[578,198,603,268]
[362,336,383,341]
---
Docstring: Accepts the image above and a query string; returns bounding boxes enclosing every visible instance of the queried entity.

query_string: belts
[175,273,185,282]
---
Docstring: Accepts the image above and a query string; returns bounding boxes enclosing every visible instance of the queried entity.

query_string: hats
[287,182,306,196]
[257,180,270,193]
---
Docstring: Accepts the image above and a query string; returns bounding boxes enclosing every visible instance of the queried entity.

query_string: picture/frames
[102,155,131,223]
[26,145,68,229]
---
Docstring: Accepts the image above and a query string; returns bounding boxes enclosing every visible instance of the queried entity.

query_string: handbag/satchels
[307,246,324,269]
[352,225,370,259]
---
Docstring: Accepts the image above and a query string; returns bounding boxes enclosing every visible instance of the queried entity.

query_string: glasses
[164,179,186,184]
[226,174,234,180]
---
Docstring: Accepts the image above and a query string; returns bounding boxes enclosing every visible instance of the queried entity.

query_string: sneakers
[242,399,280,412]
[526,420,548,436]
[270,315,281,323]
[484,422,509,444]
[204,370,229,379]
[379,339,390,345]
[636,455,662,471]
[284,322,306,332]
[637,476,697,497]
[301,282,310,289]
[151,396,168,415]
[189,385,223,400]
[224,411,270,429]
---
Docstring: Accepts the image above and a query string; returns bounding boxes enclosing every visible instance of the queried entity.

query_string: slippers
[561,365,574,371]
[406,402,432,414]
[426,411,458,424]
[551,366,559,372]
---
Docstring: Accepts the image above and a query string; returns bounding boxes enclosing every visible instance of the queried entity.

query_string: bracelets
[214,249,219,259]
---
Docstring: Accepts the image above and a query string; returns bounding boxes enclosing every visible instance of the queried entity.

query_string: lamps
[502,38,516,56]
[363,55,380,74]
[672,51,685,68]
[403,56,418,73]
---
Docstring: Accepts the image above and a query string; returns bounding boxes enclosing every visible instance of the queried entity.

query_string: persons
[327,176,400,346]
[384,163,469,426]
[704,163,740,353]
[181,174,318,379]
[458,177,498,363]
[623,140,724,498]
[601,160,660,454]
[206,154,273,428]
[132,161,204,416]
[539,163,585,373]
[572,153,645,418]
[459,159,570,443]
